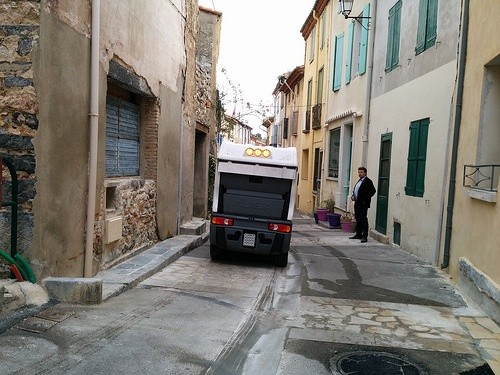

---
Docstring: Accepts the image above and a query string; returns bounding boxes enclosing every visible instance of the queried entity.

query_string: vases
[327,213,342,227]
[341,221,353,233]
[316,208,331,221]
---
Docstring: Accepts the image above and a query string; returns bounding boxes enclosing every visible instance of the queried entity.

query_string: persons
[349,167,376,243]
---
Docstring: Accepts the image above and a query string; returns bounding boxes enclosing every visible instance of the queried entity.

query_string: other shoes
[361,236,367,243]
[349,234,362,239]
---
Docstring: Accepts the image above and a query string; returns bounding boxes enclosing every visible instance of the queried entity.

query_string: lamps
[338,0,372,31]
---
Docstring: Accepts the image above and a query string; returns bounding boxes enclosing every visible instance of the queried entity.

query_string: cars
[209,139,298,269]
